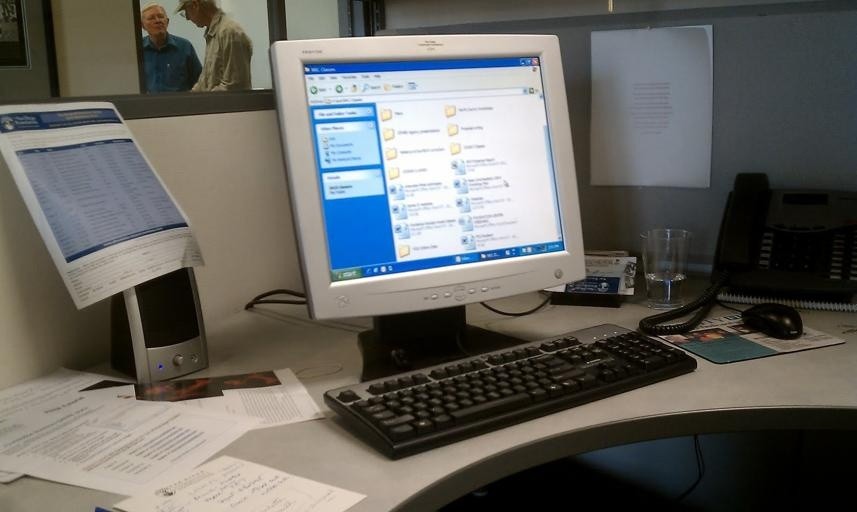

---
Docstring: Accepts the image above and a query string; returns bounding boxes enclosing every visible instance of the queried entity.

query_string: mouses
[741,301,804,340]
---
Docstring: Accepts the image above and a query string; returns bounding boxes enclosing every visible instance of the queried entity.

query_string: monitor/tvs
[267,38,588,386]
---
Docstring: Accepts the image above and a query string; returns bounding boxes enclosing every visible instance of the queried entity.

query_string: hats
[173,0,191,15]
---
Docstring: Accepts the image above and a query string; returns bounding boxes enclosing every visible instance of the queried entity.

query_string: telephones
[710,172,857,304]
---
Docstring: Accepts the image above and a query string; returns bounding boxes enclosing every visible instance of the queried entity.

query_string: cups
[639,227,693,312]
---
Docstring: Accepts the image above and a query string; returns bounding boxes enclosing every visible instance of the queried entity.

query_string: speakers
[112,267,209,385]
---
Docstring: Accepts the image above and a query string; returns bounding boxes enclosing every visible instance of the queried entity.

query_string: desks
[0,286,857,512]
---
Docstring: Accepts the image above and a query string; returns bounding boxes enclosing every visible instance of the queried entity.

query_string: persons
[141,0,203,93]
[173,0,254,92]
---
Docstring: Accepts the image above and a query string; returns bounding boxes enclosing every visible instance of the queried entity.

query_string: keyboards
[322,322,698,462]
